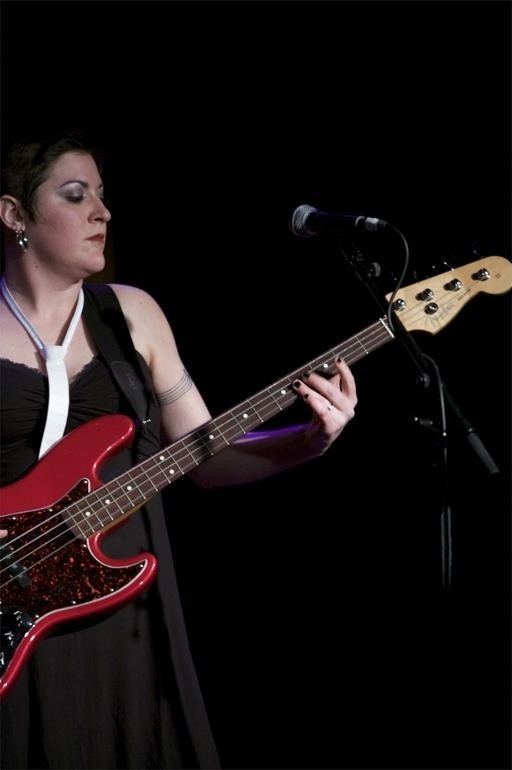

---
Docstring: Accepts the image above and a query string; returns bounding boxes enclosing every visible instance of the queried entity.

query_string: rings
[327,400,333,413]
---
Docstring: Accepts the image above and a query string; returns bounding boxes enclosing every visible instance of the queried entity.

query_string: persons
[0,138,360,770]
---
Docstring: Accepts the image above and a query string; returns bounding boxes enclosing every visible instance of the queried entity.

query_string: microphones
[291,203,387,236]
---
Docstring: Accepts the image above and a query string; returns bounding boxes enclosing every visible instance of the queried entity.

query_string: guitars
[0,254,512,700]
